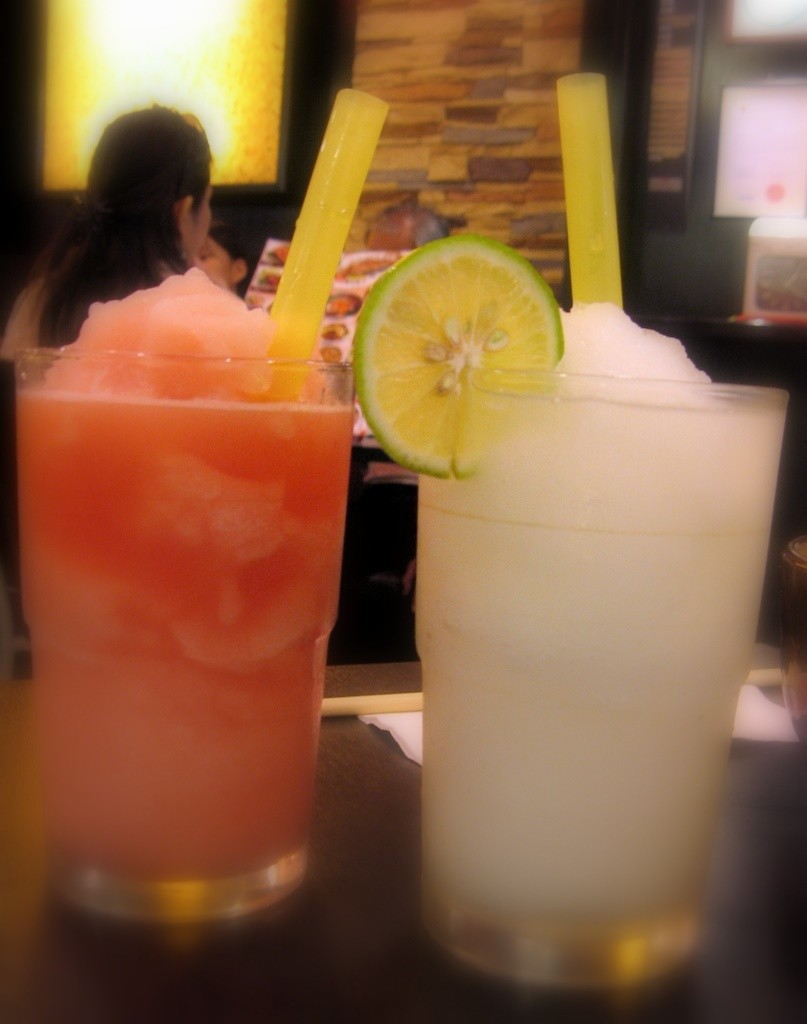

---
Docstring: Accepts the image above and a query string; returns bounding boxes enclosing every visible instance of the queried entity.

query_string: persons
[0,103,267,362]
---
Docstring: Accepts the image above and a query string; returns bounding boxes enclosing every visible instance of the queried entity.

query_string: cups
[417,361,796,999]
[14,343,355,930]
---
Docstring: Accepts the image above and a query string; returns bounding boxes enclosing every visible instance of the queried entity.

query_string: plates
[326,294,361,316]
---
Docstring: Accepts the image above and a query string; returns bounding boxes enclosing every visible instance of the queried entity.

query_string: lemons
[350,233,563,480]
